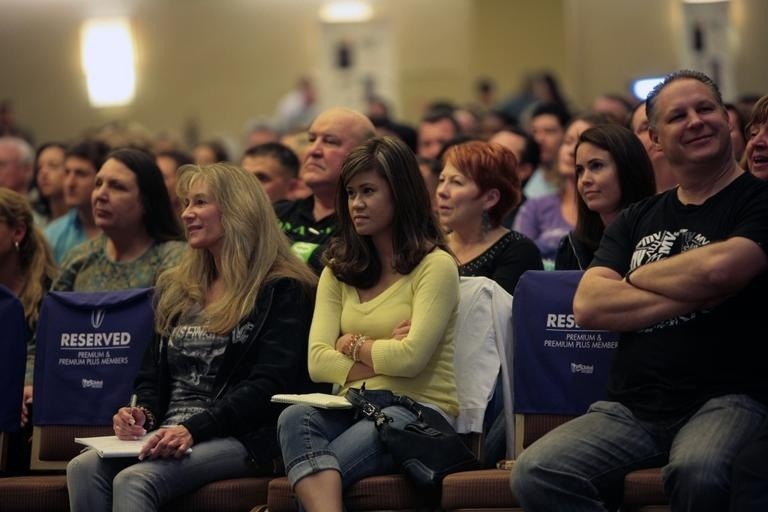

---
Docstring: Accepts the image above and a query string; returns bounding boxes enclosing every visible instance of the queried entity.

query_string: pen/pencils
[129,395,137,415]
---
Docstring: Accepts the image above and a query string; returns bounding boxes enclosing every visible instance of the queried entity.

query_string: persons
[1,71,768,510]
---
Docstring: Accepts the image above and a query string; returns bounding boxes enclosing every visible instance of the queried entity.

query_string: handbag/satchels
[375,396,473,489]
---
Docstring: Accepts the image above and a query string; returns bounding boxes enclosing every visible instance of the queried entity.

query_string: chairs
[0,269,768,512]
